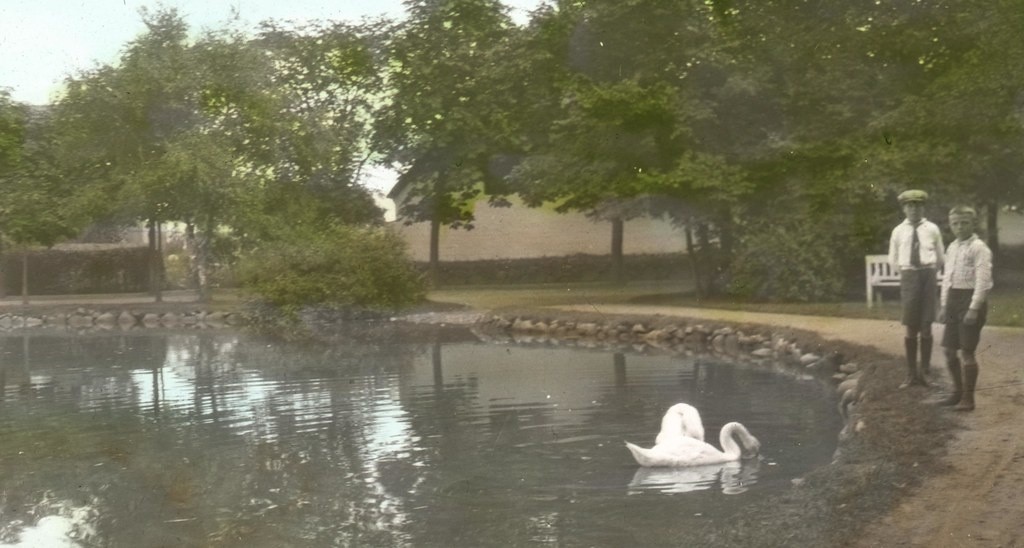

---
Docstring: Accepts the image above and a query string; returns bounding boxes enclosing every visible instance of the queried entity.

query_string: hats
[897,189,930,205]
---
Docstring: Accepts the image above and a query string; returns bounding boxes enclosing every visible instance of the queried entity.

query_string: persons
[938,205,995,412]
[886,188,946,391]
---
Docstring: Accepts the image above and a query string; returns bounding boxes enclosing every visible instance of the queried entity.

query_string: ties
[908,222,923,268]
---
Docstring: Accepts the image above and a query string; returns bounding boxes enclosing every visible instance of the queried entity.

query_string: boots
[937,360,963,406]
[919,333,942,390]
[949,363,978,412]
[898,335,919,390]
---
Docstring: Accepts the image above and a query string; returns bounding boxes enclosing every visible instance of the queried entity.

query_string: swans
[624,403,762,468]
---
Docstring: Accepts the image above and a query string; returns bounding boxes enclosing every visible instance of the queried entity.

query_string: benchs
[864,255,952,307]
